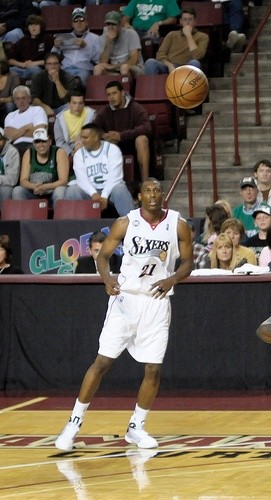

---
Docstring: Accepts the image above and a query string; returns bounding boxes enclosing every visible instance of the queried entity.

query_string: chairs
[1,199,49,219]
[86,74,132,112]
[53,200,102,218]
[133,75,180,153]
[122,154,136,196]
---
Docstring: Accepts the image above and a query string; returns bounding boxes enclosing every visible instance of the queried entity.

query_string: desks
[0,273,271,397]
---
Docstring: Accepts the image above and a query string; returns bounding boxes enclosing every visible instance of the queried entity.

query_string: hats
[240,176,257,188]
[32,128,49,141]
[252,206,271,220]
[105,10,121,24]
[72,8,86,19]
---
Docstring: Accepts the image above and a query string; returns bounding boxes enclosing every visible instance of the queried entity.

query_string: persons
[54,179,193,451]
[0,81,156,217]
[75,231,122,274]
[192,159,271,273]
[256,316,271,344]
[0,235,24,275]
[0,0,264,130]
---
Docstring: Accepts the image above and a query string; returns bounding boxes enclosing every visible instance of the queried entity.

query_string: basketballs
[164,65,209,109]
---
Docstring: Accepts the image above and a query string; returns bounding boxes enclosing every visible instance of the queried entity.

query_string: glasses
[74,17,85,23]
[34,140,47,143]
[46,62,60,65]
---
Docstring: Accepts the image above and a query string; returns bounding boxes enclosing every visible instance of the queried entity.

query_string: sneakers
[125,448,159,469]
[54,415,83,451]
[56,460,83,483]
[125,423,159,449]
[227,30,239,49]
[235,33,246,53]
